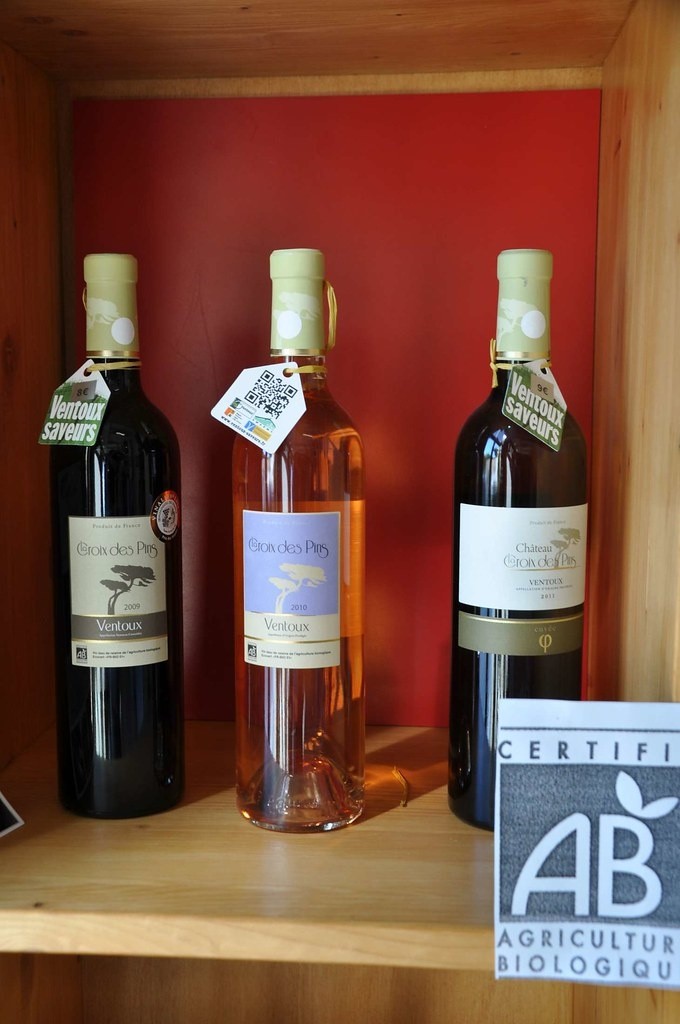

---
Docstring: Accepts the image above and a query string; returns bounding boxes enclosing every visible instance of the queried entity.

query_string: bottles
[48,254,186,821]
[449,247,588,832]
[230,245,367,836]
